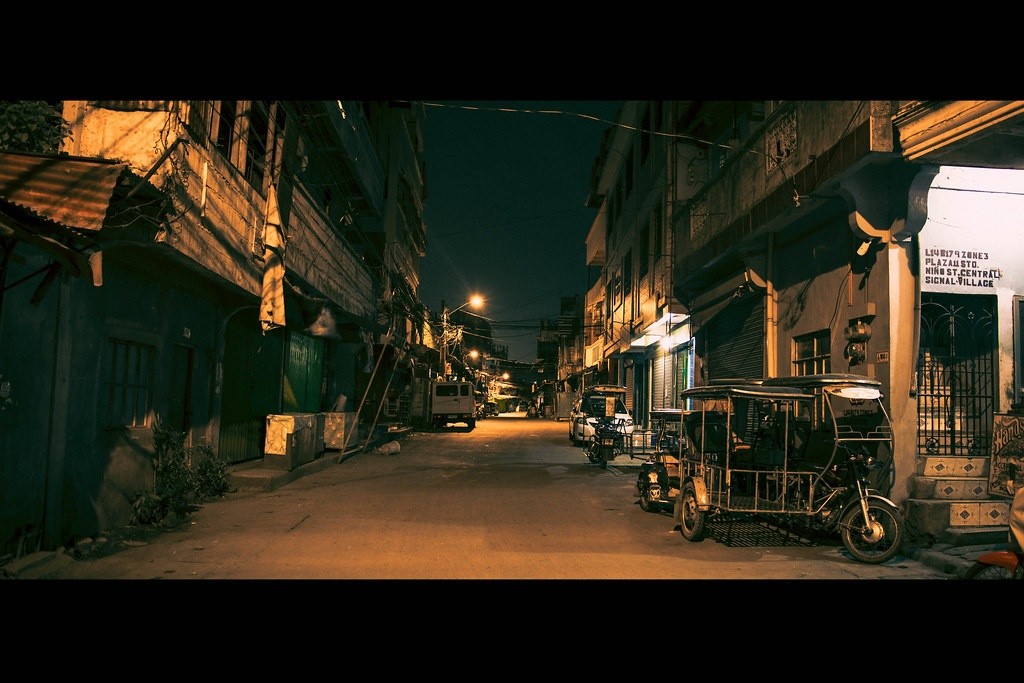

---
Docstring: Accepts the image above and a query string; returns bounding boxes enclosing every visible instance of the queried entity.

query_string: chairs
[760,410,850,498]
[686,410,756,494]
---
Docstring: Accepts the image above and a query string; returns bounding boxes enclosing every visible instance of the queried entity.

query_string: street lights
[440,294,486,362]
[492,372,509,383]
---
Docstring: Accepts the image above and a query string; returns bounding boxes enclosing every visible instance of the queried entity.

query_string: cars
[569,395,635,445]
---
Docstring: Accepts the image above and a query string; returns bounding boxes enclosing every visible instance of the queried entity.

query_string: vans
[487,402,501,417]
[432,381,477,428]
[518,400,528,412]
[586,385,627,409]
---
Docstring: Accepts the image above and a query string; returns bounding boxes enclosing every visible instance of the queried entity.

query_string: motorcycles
[637,373,905,564]
[588,414,616,470]
